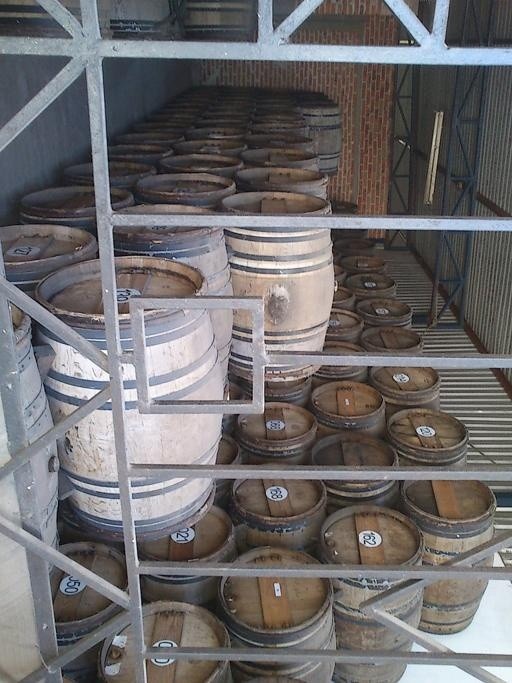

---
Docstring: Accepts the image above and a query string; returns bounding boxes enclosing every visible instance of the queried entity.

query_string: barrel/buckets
[0,1,262,40]
[0,85,499,683]
